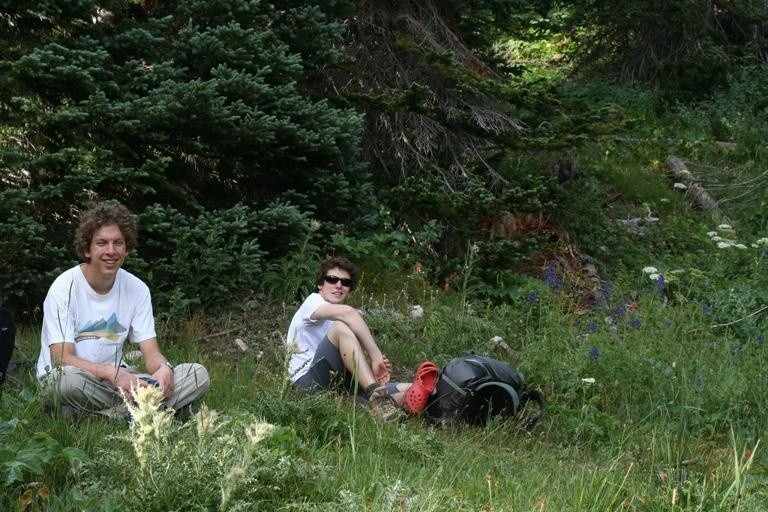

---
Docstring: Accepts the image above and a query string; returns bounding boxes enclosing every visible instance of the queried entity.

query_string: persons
[284,255,440,427]
[32,200,212,428]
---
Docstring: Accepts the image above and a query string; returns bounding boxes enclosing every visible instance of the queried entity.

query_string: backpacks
[428,353,544,428]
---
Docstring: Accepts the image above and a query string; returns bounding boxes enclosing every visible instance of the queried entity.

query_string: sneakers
[172,401,200,424]
[369,386,409,423]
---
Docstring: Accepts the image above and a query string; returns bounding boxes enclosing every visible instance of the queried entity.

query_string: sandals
[403,361,439,415]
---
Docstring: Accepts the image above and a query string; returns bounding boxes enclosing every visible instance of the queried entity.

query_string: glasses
[325,274,352,287]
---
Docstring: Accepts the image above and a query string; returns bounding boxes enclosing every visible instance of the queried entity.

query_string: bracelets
[156,359,176,373]
